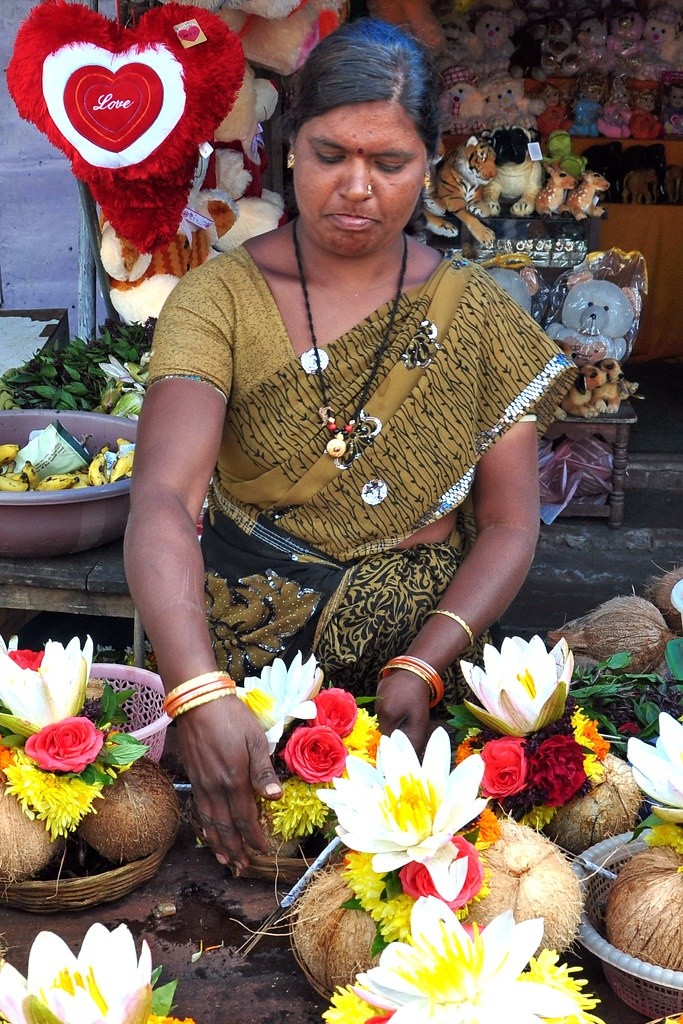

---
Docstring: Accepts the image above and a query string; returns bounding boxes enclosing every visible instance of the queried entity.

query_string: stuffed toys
[101,0,683,418]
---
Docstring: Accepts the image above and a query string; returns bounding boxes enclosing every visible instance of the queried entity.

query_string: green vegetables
[1,317,159,421]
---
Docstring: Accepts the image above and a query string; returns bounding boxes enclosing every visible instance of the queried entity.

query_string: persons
[122,17,580,874]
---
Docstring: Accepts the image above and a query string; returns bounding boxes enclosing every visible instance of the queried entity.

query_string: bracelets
[421,609,476,647]
[379,654,444,708]
[164,671,237,720]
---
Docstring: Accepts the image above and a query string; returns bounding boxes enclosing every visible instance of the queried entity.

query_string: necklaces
[293,216,407,457]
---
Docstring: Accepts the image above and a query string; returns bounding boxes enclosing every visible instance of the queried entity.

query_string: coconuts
[0,755,181,883]
[244,565,683,999]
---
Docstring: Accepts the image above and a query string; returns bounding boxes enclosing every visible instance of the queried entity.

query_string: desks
[0,539,146,667]
[543,399,637,527]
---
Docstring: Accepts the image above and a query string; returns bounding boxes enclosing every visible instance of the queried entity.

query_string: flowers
[0,315,156,422]
[1,631,157,832]
[235,634,683,1024]
[0,922,195,1024]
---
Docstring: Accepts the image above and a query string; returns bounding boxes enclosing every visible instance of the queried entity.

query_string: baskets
[0,822,178,913]
[570,829,683,1022]
[78,663,173,763]
[246,852,317,884]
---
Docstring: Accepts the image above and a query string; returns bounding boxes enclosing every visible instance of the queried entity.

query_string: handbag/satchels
[201,469,347,687]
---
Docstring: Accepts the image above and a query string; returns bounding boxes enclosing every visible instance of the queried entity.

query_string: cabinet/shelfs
[426,73,683,370]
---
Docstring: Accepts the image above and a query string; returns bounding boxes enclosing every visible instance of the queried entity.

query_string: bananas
[0,436,141,493]
